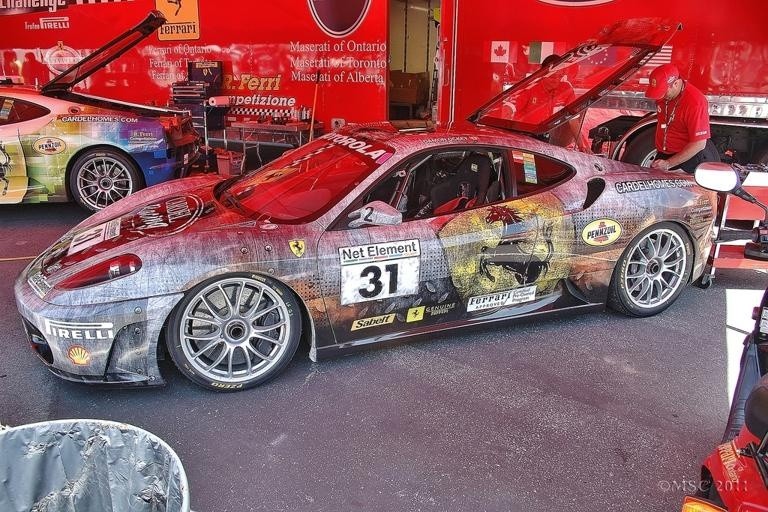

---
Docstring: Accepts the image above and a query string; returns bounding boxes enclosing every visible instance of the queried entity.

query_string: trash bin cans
[0,419,190,512]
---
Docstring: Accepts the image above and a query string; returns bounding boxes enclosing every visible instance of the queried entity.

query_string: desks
[389,102,414,121]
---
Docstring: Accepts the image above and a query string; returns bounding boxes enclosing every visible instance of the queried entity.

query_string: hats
[645,64,679,100]
[734,384,767,452]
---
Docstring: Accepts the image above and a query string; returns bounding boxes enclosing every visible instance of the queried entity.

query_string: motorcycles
[682,161,768,510]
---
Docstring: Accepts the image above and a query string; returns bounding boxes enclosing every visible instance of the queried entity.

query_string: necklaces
[662,81,684,150]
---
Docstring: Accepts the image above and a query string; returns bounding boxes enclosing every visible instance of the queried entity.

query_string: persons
[3,50,50,86]
[645,63,713,174]
[511,54,593,154]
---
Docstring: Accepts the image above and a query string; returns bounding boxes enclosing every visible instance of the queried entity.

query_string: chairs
[366,151,500,221]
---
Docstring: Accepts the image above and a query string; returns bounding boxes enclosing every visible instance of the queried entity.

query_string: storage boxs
[217,153,246,176]
[389,70,429,105]
